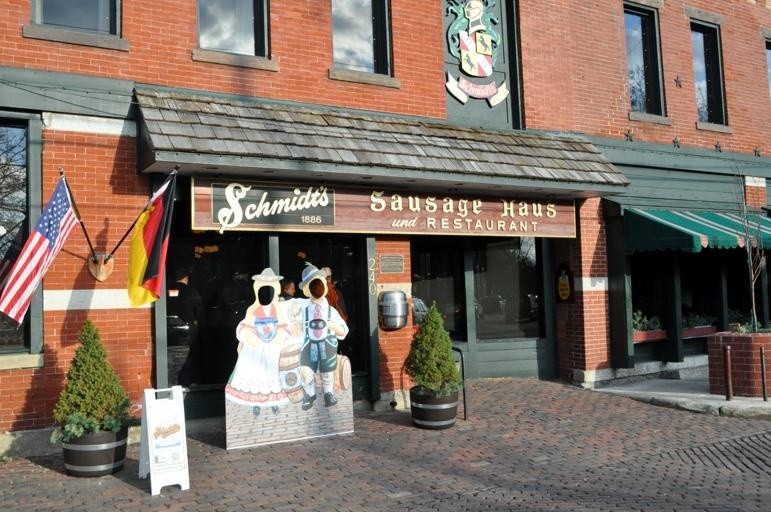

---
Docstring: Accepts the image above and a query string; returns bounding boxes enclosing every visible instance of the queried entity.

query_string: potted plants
[406,299,465,428]
[682,310,717,339]
[51,318,138,479]
[632,310,668,345]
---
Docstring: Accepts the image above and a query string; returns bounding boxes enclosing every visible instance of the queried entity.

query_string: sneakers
[302,393,316,409]
[325,393,337,407]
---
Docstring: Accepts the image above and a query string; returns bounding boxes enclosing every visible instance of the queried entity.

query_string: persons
[280,280,296,300]
[167,267,201,387]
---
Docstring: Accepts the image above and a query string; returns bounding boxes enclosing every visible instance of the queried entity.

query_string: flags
[126,173,177,308]
[0,178,78,331]
